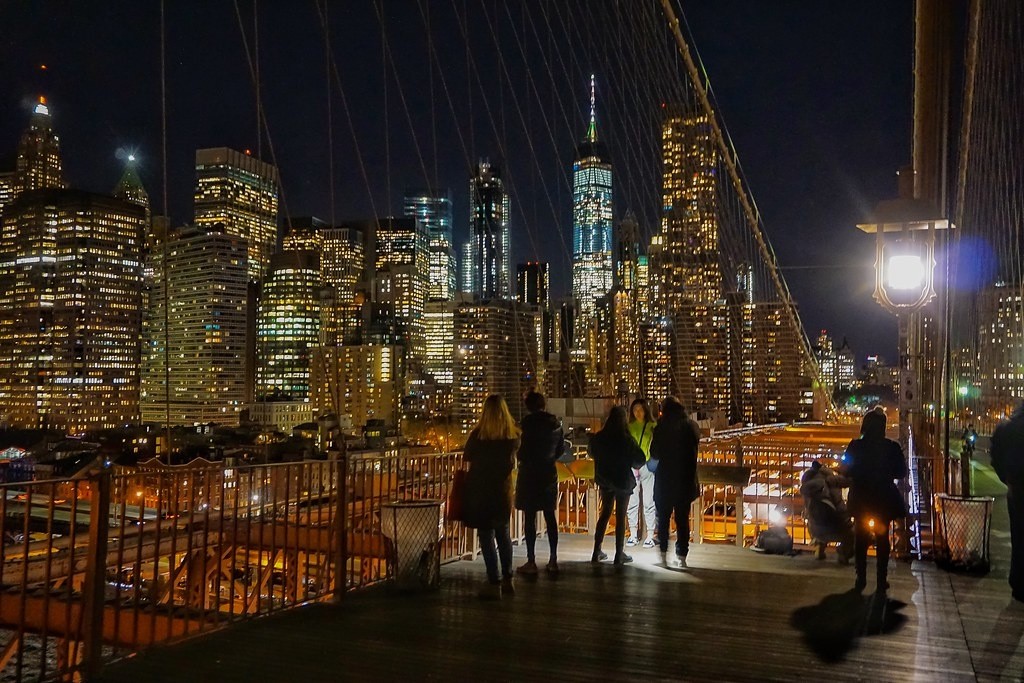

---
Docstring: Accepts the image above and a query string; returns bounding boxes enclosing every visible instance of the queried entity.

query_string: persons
[626,397,660,549]
[461,393,523,604]
[843,404,908,592]
[648,396,702,569]
[990,398,1024,603]
[961,423,979,454]
[513,392,566,577]
[586,404,646,566]
[798,460,854,568]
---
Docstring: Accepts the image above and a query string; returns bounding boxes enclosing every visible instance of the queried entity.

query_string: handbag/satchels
[450,469,471,520]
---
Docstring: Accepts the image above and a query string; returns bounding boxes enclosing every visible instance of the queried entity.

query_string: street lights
[857,166,956,521]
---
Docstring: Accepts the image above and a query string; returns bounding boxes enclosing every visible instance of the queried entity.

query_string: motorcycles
[962,434,978,459]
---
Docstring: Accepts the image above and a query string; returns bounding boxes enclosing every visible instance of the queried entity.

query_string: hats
[861,406,886,435]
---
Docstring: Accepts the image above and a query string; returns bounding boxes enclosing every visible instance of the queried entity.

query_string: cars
[4,511,351,616]
[779,487,804,515]
[774,470,805,488]
[701,501,752,540]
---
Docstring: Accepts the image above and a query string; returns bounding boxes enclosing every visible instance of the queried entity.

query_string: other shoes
[650,552,687,568]
[593,549,633,565]
[482,576,515,598]
[814,543,826,559]
[836,553,849,563]
[517,559,559,576]
[855,572,890,590]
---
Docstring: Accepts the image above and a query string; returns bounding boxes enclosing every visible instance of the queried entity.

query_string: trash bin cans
[378,497,445,594]
[935,491,996,576]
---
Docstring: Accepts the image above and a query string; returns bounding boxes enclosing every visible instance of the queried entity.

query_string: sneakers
[643,538,655,547]
[626,535,639,546]
